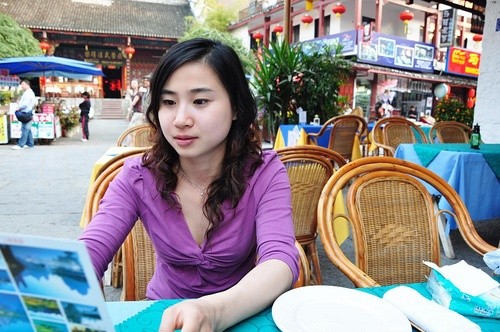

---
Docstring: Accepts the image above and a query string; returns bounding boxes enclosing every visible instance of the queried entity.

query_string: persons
[9,80,35,149]
[79,92,92,142]
[141,73,155,125]
[127,79,143,139]
[375,90,419,121]
[76,37,299,332]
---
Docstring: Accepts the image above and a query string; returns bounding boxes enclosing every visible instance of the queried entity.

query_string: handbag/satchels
[382,286,480,332]
[79,118,82,122]
[16,110,32,123]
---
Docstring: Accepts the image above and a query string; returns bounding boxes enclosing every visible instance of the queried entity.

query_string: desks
[275,124,437,161]
[106,273,500,332]
[394,143,500,234]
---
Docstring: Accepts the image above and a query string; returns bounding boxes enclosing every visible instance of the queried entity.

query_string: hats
[143,73,153,80]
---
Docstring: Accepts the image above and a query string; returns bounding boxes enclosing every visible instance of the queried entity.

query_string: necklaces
[177,168,210,196]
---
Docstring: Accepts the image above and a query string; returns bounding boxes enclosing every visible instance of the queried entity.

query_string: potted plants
[246,34,306,147]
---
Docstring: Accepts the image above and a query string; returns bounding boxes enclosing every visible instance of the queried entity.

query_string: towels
[382,285,481,332]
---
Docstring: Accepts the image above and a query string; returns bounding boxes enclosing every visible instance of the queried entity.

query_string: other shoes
[83,139,87,142]
[23,145,30,149]
[80,137,83,140]
[11,145,23,149]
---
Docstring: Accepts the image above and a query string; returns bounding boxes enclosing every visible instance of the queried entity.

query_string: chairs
[86,106,497,301]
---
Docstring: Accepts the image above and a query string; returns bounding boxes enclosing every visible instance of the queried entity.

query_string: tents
[302,15,313,27]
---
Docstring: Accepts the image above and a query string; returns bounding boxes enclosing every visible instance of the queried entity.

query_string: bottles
[314,114,320,126]
[471,124,481,149]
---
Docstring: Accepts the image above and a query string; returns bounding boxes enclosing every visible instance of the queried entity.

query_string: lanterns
[467,98,474,109]
[40,41,51,54]
[473,33,483,43]
[332,4,346,17]
[124,47,136,59]
[399,10,414,24]
[252,33,264,43]
[468,89,475,98]
[273,26,284,36]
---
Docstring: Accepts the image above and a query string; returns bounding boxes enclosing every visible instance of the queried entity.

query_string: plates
[409,321,424,332]
[271,285,412,332]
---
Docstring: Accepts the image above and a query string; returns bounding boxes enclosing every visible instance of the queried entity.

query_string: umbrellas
[0,55,105,102]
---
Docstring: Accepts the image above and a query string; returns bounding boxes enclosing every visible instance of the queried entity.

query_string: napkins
[383,286,482,332]
